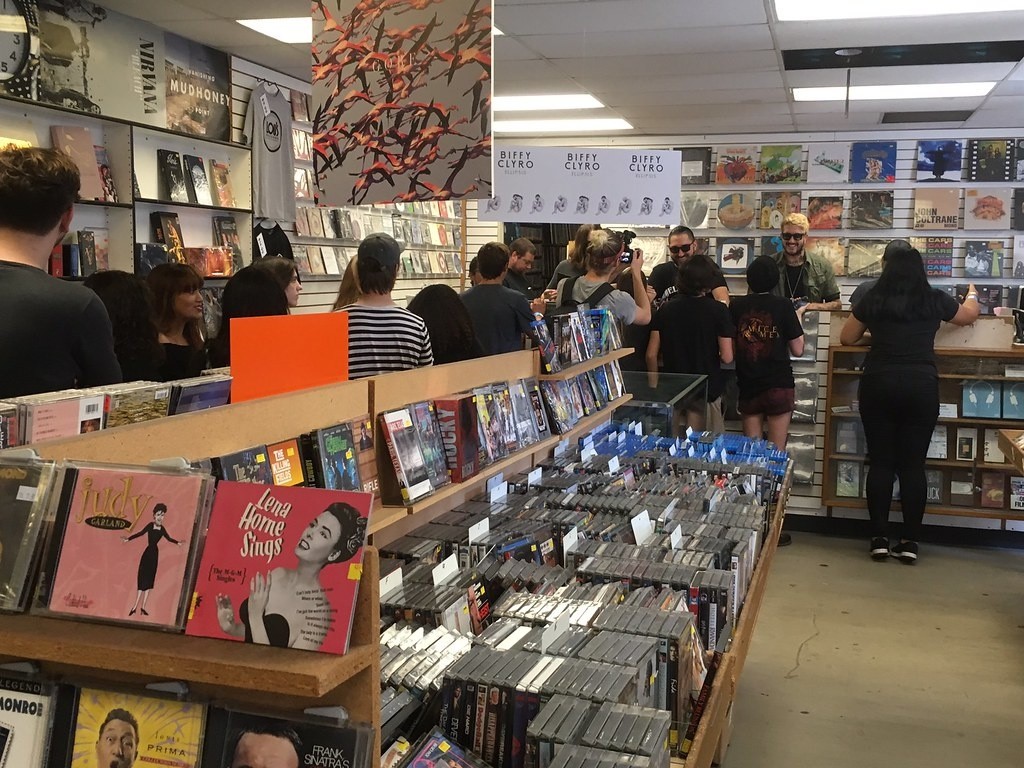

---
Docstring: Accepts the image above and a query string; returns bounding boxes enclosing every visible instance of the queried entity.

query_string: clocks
[0,0,31,82]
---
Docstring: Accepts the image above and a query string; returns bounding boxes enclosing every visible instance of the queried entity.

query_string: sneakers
[868,536,890,557]
[888,542,919,561]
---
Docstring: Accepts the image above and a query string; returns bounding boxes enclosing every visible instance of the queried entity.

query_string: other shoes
[778,533,792,546]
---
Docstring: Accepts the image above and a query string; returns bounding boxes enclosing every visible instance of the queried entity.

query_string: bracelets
[966,291,978,302]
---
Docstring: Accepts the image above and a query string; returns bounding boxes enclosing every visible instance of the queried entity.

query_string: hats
[357,233,407,271]
[679,254,722,289]
[747,255,780,293]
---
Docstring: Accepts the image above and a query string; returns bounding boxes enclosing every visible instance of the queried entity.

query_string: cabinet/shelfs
[0,94,252,279]
[0,350,795,768]
[822,346,1024,521]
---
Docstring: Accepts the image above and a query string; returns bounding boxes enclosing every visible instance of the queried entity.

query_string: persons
[360,422,373,451]
[489,687,499,705]
[216,502,365,651]
[458,224,647,372]
[84,232,487,380]
[467,586,483,636]
[96,709,139,768]
[670,642,678,661]
[646,254,734,433]
[727,255,810,547]
[839,240,979,563]
[226,722,303,768]
[0,143,123,400]
[965,245,990,277]
[454,684,465,711]
[747,213,842,311]
[647,226,730,308]
[330,458,358,491]
[437,754,462,768]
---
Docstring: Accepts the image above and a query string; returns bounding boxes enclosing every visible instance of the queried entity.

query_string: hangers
[255,77,281,92]
[253,217,284,232]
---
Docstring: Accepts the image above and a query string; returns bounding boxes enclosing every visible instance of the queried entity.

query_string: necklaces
[785,265,803,303]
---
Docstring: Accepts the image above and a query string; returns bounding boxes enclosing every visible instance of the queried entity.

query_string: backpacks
[541,277,616,341]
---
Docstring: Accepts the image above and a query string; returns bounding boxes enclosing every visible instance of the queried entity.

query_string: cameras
[621,249,634,263]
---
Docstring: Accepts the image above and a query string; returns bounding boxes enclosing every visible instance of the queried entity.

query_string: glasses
[781,232,805,241]
[669,241,695,253]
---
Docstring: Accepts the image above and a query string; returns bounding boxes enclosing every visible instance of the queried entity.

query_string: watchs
[534,312,543,317]
